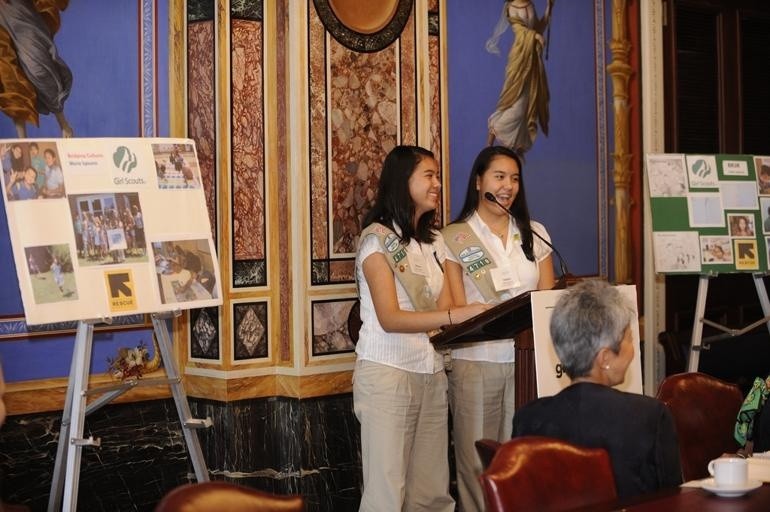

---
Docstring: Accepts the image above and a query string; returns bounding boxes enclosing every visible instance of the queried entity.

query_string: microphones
[485,191,570,275]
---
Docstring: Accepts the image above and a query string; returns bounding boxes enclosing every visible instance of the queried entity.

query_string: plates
[699,476,769,497]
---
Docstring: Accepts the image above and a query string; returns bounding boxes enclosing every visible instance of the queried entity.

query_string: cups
[753,454,770,477]
[706,457,746,487]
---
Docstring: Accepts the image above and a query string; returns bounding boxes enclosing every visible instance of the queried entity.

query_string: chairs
[156,482,303,512]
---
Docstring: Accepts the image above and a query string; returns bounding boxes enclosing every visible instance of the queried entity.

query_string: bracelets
[446,307,454,326]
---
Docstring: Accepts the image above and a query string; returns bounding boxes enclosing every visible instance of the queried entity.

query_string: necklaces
[486,222,509,237]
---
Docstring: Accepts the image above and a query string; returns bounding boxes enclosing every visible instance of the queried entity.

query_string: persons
[349,141,497,512]
[72,204,146,264]
[719,375,770,458]
[49,256,65,294]
[487,0,552,147]
[155,144,194,185]
[2,141,65,199]
[28,254,46,279]
[510,277,683,497]
[736,216,752,236]
[0,0,73,138]
[440,145,558,512]
